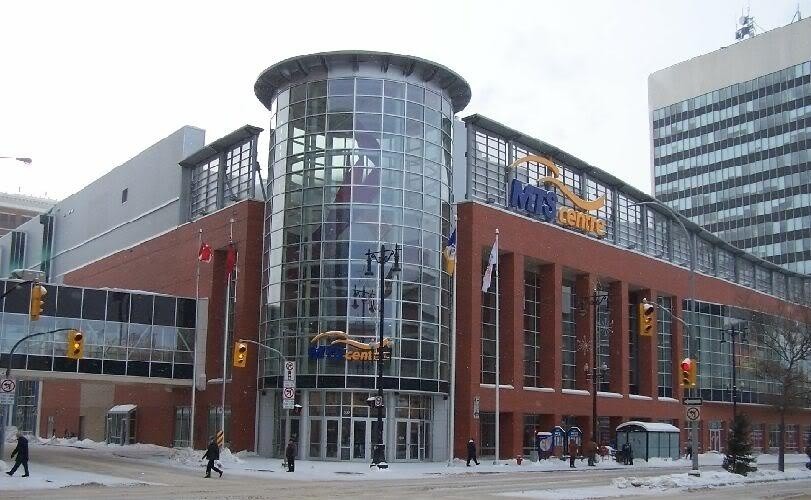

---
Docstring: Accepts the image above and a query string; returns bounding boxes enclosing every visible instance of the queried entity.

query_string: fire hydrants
[515,455,523,466]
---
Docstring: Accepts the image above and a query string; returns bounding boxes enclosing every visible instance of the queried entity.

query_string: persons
[465,438,481,467]
[5,432,30,478]
[285,438,296,473]
[569,439,577,468]
[686,438,693,460]
[201,435,223,479]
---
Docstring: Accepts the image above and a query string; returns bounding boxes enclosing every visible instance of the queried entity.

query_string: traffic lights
[678,358,697,388]
[233,342,247,368]
[68,330,83,359]
[639,303,657,336]
[31,286,46,321]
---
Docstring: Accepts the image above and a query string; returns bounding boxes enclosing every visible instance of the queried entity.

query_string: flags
[442,214,457,276]
[481,240,497,293]
[197,242,213,263]
[223,241,236,284]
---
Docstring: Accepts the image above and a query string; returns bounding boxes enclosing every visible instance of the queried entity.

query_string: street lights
[578,289,610,466]
[365,243,402,468]
[351,285,376,372]
[719,326,748,415]
